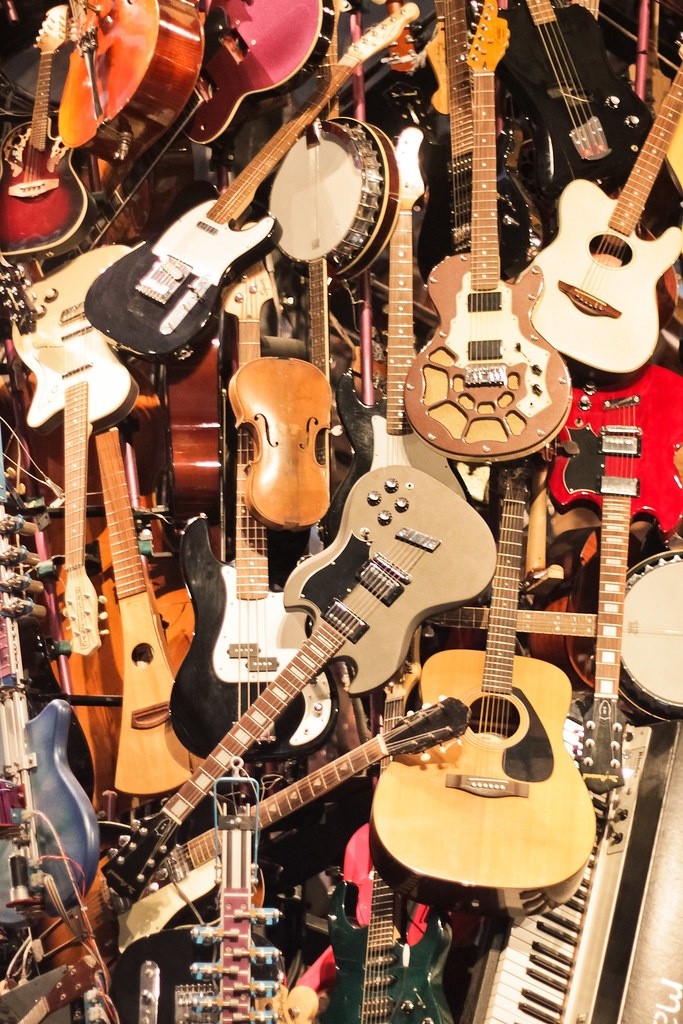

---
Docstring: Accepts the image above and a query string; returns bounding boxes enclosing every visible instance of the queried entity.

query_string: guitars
[100,465,498,914]
[366,458,597,916]
[0,0,683,1024]
[403,0,573,463]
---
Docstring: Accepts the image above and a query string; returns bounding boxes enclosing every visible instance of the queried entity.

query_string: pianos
[458,690,683,1023]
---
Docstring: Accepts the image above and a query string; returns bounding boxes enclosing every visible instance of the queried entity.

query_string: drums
[268,115,400,281]
[616,550,683,721]
